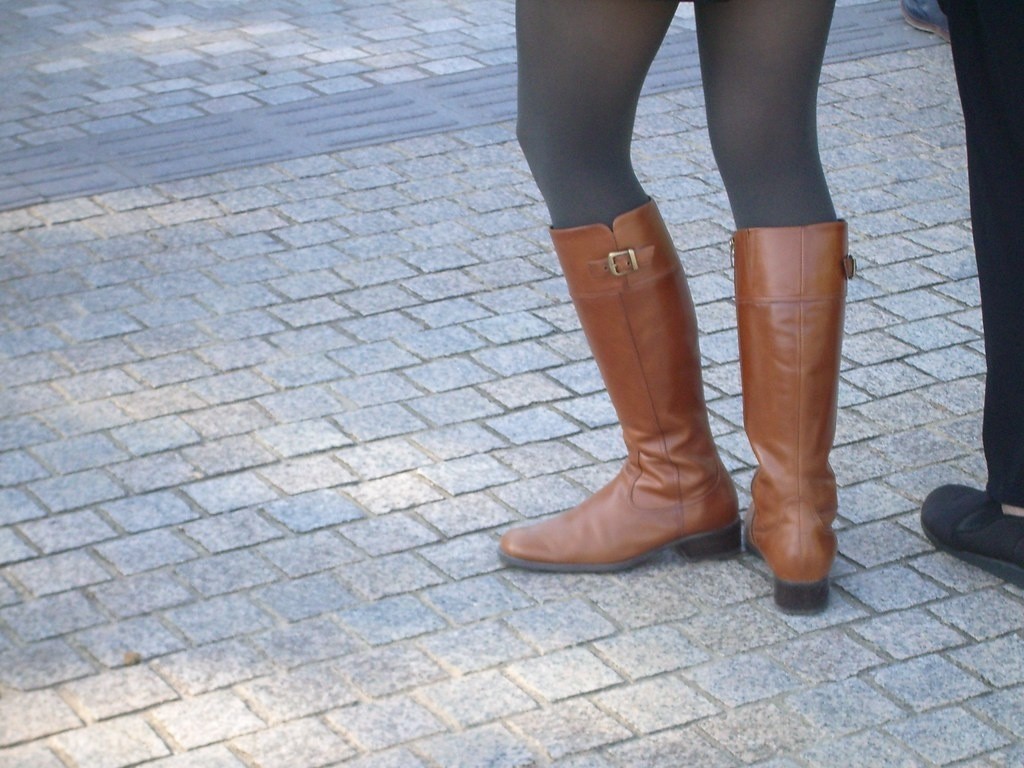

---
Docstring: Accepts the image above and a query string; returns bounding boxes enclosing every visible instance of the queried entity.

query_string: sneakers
[920,483,1024,587]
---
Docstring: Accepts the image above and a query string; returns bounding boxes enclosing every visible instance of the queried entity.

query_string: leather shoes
[899,0,951,43]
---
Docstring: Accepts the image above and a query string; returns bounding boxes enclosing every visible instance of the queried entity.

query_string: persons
[496,0,858,616]
[893,0,1024,592]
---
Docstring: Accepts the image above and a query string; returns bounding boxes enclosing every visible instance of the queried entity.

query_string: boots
[727,218,856,615]
[495,197,743,575]
